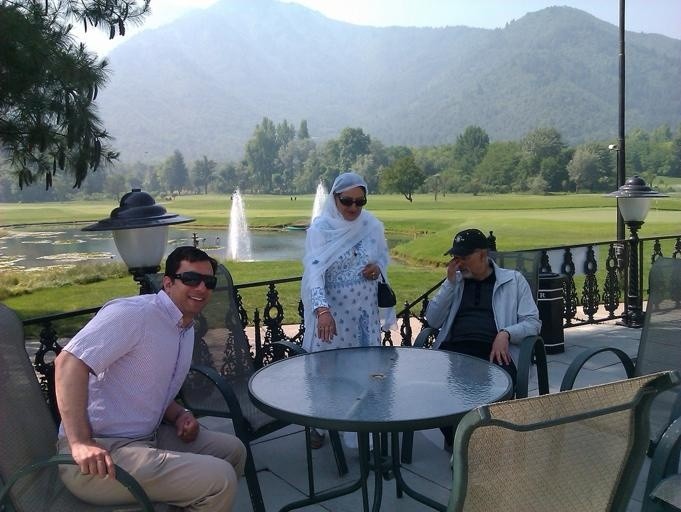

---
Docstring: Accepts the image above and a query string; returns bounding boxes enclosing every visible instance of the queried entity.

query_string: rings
[372,272,375,276]
[372,276,375,280]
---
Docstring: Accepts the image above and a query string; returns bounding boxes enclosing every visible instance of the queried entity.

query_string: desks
[247,346,514,511]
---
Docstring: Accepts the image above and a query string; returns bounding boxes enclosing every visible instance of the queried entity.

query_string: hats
[444,229,488,256]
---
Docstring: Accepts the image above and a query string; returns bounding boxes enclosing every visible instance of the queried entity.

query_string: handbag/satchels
[378,282,396,308]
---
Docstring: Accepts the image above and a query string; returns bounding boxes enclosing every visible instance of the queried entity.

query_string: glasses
[338,195,366,206]
[171,271,217,289]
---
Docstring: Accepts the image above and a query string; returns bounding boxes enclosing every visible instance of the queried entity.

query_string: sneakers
[310,429,324,449]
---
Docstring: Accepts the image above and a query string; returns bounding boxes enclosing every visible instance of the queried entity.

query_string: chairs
[3,301,174,511]
[559,255,680,459]
[447,370,680,511]
[400,252,551,466]
[143,260,349,511]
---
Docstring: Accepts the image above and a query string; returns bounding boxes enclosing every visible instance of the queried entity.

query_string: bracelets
[499,329,511,342]
[174,408,192,426]
[318,310,329,319]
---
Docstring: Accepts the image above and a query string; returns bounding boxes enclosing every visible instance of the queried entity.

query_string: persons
[300,173,397,471]
[427,229,543,453]
[54,246,247,512]
